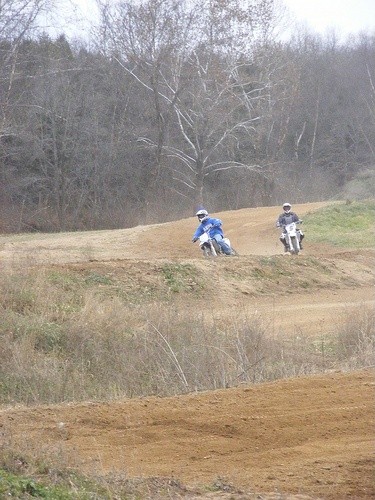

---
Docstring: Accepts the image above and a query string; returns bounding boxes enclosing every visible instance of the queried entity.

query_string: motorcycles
[192,223,239,257]
[277,220,304,255]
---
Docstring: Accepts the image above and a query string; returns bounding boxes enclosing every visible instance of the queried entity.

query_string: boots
[280,238,289,252]
[299,234,304,249]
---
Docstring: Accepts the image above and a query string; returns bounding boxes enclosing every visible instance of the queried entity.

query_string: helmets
[196,210,209,223]
[283,203,292,214]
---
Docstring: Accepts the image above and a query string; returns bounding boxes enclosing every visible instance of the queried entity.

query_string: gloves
[213,223,218,228]
[192,238,196,243]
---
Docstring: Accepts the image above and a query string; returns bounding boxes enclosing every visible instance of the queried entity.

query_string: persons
[192,210,232,254]
[276,203,304,252]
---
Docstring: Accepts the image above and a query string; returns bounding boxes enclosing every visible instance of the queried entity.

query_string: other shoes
[228,252,236,256]
